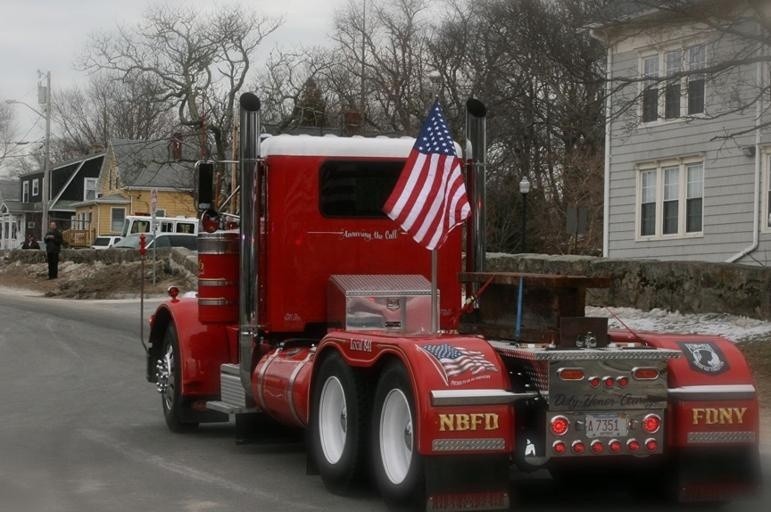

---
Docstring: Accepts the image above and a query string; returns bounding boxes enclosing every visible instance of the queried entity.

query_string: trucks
[129,92,760,511]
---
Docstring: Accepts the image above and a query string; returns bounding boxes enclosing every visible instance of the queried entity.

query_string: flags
[380,94,474,251]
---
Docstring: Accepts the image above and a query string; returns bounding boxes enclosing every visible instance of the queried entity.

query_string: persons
[21,233,43,251]
[44,220,64,281]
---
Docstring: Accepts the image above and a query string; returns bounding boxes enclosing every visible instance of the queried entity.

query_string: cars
[90,213,198,265]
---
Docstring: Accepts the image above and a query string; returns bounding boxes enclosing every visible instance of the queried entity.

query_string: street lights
[517,171,533,253]
[7,67,53,245]
